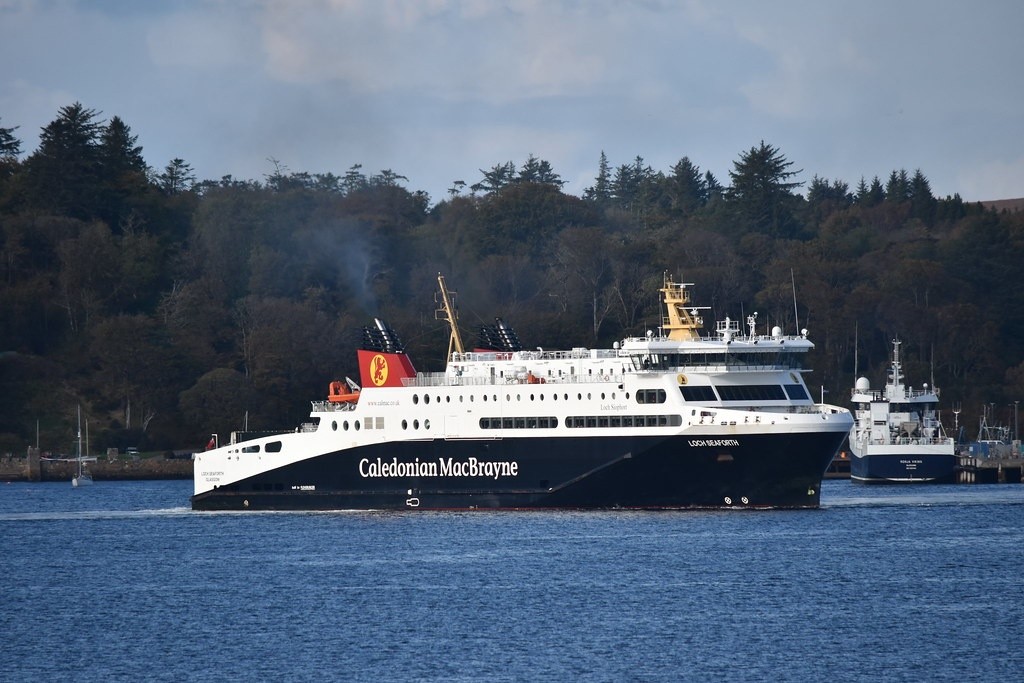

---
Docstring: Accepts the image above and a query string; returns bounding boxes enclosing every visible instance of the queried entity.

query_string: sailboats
[72,404,93,486]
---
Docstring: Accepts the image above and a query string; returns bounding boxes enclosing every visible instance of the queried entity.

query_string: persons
[644,357,650,369]
[527,370,535,384]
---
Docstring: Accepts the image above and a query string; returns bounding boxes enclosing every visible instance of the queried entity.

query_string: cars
[125,447,141,454]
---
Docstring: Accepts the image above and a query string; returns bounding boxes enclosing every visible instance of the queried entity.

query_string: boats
[849,342,954,485]
[191,274,855,511]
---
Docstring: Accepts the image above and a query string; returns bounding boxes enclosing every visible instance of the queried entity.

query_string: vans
[978,440,1005,445]
